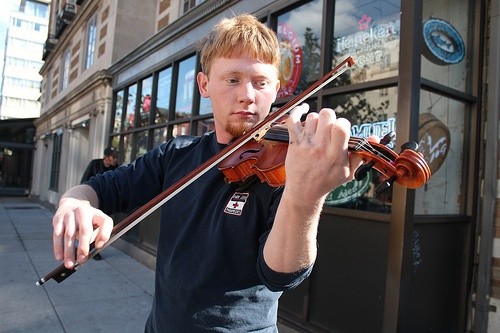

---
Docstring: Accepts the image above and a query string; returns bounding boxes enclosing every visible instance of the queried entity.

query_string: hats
[104,147,116,156]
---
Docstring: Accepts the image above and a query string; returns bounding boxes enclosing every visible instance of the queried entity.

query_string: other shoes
[90,249,101,260]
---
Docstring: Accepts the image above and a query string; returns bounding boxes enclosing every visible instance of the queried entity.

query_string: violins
[216,112,431,194]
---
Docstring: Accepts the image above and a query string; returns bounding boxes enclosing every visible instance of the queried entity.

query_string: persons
[51,13,380,333]
[74,146,121,260]
